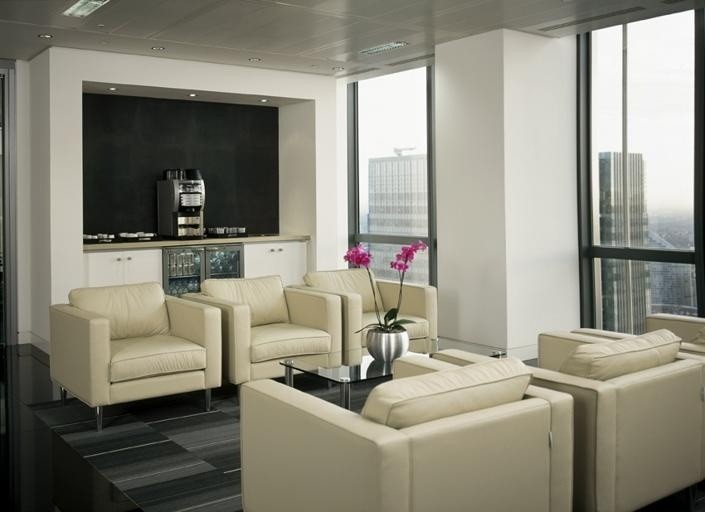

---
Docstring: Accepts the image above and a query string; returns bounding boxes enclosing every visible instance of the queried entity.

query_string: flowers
[342,238,429,334]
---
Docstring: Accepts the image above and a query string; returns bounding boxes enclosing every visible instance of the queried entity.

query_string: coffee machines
[156,167,207,241]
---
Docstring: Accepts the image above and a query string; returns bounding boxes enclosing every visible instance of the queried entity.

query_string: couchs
[571,313,705,356]
[432,330,705,512]
[180,274,342,403]
[239,352,576,511]
[286,266,438,384]
[49,282,222,432]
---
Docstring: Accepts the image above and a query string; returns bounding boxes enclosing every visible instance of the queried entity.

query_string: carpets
[21,374,394,512]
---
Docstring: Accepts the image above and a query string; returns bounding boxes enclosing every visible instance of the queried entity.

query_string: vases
[366,329,409,363]
[366,358,391,378]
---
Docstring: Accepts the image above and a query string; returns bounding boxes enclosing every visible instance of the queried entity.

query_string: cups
[169,280,199,296]
[169,252,197,277]
[208,227,246,234]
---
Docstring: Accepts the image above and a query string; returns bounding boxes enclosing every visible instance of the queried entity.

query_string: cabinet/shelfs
[162,242,244,299]
[244,241,307,288]
[83,247,162,286]
